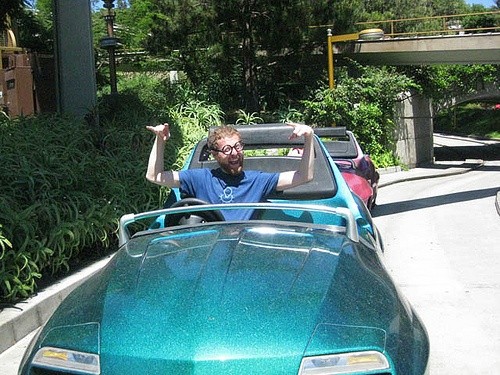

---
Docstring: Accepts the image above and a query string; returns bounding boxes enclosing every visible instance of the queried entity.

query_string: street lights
[327,25,386,128]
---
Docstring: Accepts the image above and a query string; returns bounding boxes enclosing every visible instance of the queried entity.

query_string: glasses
[212,141,245,156]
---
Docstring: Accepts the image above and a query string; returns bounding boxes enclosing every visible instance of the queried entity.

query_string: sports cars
[307,125,381,213]
[17,123,430,375]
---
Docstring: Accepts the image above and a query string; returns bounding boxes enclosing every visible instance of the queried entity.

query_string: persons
[144,120,315,222]
